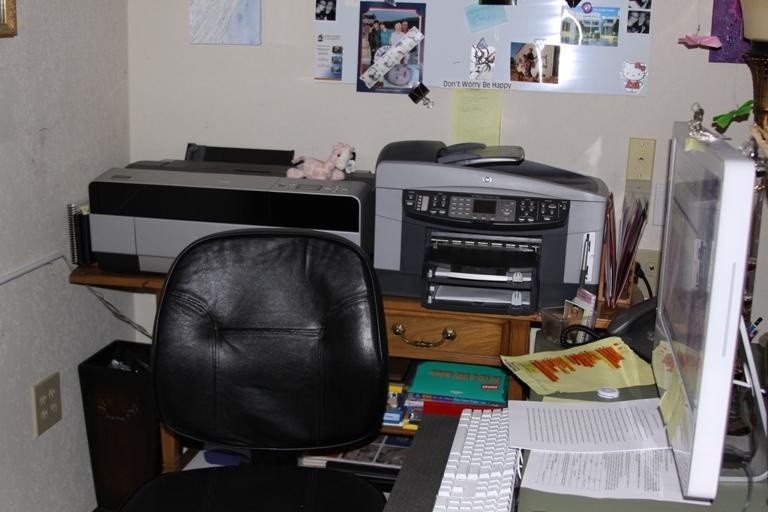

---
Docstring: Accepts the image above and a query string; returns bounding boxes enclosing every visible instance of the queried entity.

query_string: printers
[88,141,376,279]
[372,140,611,316]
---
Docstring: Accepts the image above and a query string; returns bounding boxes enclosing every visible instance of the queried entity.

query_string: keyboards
[431,408,524,512]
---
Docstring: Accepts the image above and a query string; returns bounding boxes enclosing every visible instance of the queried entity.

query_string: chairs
[116,227,389,512]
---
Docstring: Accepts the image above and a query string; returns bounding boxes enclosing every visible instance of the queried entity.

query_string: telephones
[605,296,658,361]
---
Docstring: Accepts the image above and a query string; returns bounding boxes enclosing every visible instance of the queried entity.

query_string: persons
[632,11,648,32]
[627,11,638,32]
[369,23,392,50]
[401,21,409,33]
[564,307,581,343]
[315,0,325,19]
[367,19,380,65]
[320,0,335,20]
[389,22,407,64]
[523,47,535,77]
[379,63,420,87]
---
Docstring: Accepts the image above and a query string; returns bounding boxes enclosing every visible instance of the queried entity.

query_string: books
[561,298,587,346]
[297,359,511,483]
[572,296,590,345]
[574,286,597,343]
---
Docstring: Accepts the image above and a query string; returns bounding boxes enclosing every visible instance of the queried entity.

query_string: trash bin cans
[78,339,162,510]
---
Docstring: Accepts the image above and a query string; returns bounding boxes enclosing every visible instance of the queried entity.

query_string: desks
[383,327,768,512]
[69,263,543,501]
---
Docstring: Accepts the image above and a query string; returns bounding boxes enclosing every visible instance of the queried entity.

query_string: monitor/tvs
[651,120,768,501]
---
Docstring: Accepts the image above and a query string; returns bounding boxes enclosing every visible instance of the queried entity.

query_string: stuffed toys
[285,140,357,182]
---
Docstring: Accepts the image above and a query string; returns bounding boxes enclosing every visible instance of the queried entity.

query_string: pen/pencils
[748,317,764,343]
